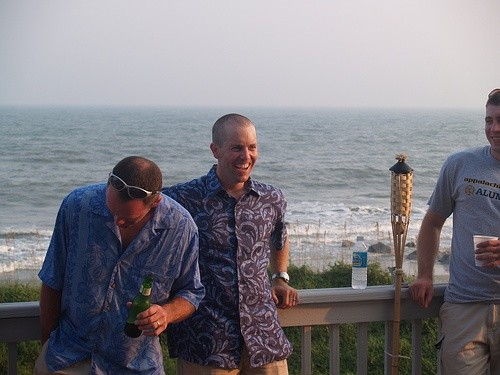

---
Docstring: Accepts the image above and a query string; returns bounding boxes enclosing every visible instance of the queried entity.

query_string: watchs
[271,272,290,282]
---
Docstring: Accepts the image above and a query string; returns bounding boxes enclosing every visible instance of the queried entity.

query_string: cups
[473,235,498,266]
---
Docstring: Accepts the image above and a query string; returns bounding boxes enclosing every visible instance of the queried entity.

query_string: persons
[407,89,500,375]
[33,155,205,375]
[159,114,299,375]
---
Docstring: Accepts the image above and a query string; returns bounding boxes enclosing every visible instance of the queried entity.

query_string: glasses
[107,171,161,201]
[487,88,500,102]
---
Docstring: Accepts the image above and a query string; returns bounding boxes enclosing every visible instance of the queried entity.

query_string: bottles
[352,236,367,289]
[124,275,153,338]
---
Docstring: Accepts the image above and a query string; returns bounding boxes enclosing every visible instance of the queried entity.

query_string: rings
[156,321,162,327]
[293,299,296,301]
[416,286,420,291]
